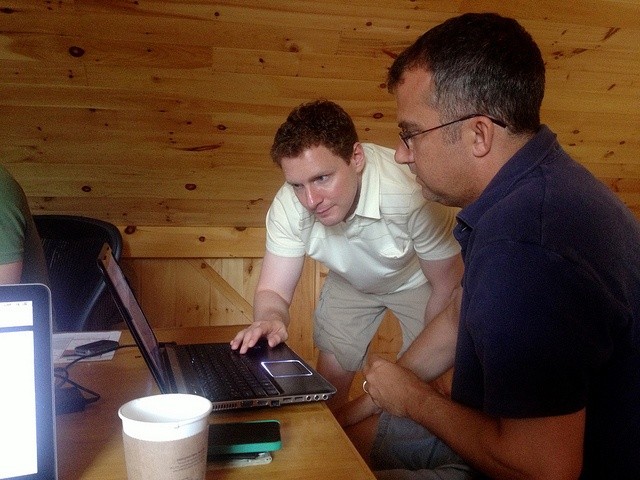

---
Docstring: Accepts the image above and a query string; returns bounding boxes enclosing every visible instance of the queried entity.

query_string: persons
[1,161,57,331]
[358,10,638,478]
[228,100,465,428]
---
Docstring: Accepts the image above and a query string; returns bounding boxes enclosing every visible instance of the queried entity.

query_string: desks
[53,323,377,480]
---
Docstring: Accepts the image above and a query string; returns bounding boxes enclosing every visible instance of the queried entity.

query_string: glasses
[399,113,507,149]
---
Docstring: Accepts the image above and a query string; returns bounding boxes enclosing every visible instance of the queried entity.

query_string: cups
[118,394,211,479]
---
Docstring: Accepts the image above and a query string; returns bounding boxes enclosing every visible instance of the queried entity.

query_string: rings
[362,380,367,395]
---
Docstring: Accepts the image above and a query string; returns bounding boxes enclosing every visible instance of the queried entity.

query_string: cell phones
[209,420,282,455]
[75,339,119,356]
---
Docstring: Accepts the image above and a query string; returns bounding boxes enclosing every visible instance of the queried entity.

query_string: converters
[56,387,85,413]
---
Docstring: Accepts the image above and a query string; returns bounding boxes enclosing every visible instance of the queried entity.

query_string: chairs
[32,213,123,333]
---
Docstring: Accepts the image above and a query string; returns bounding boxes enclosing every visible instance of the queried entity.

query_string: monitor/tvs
[0,283,59,480]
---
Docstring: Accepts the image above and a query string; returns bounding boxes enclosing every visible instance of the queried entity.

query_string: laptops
[97,243,337,413]
